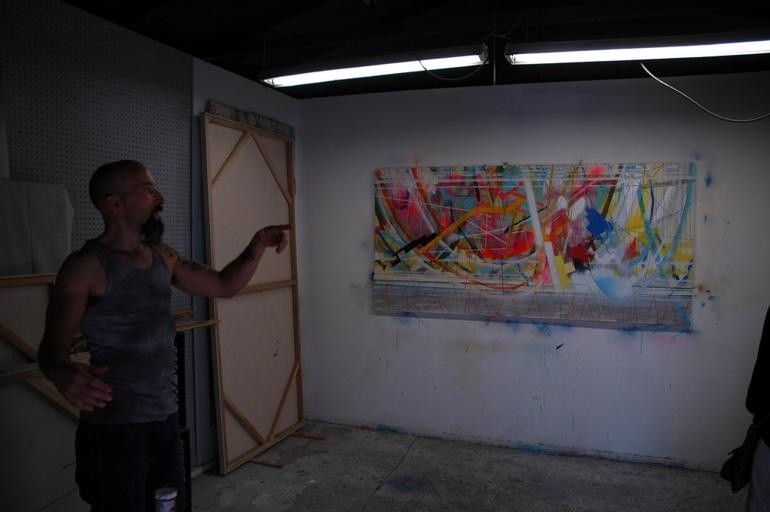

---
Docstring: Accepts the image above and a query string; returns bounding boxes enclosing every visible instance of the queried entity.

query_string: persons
[35,160,292,512]
[718,305,770,512]
